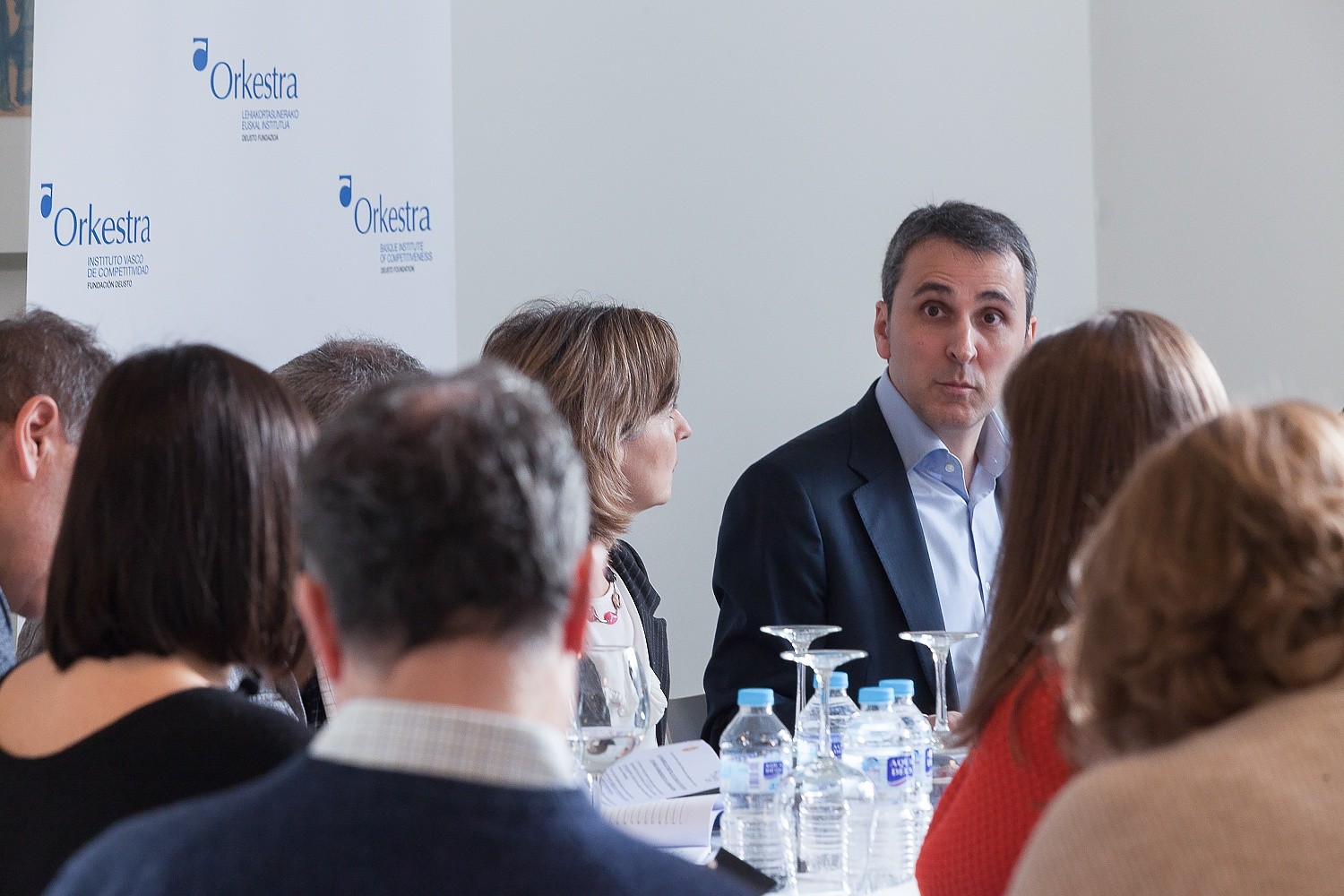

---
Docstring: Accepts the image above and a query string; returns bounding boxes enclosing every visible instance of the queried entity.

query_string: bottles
[842,686,916,888]
[879,677,934,859]
[719,687,797,887]
[795,671,860,862]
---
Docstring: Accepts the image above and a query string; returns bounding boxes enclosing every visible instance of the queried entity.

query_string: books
[599,795,724,867]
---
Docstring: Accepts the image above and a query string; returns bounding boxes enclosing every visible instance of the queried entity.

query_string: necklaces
[588,567,620,624]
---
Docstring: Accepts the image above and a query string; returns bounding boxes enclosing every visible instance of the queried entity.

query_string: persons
[37,354,756,896]
[1006,399,1344,896]
[272,340,429,730]
[913,312,1231,896]
[0,345,322,895]
[0,309,112,681]
[703,200,1037,759]
[479,298,692,746]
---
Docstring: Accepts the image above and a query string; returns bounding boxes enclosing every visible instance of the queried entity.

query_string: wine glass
[570,644,651,812]
[779,646,872,893]
[899,630,980,807]
[759,625,843,716]
